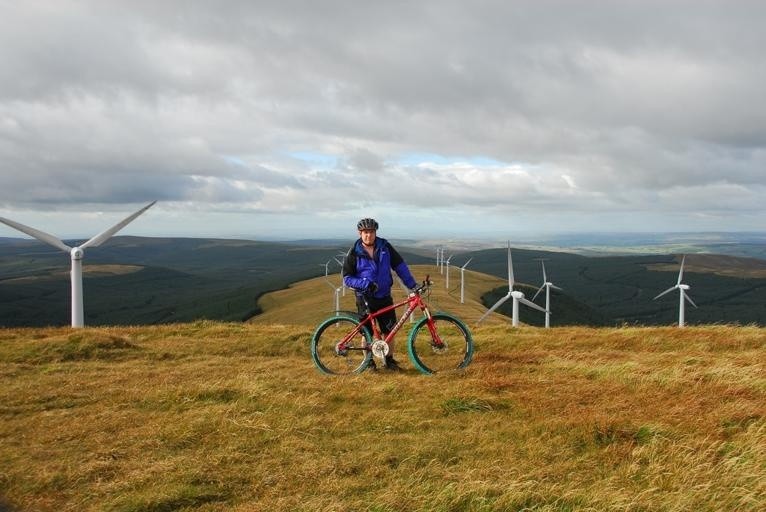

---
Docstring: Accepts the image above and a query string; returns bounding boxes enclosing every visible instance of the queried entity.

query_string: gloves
[366,282,378,293]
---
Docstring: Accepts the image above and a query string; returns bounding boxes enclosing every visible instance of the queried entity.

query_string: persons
[342,218,426,372]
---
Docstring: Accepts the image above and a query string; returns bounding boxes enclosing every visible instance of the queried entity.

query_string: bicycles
[309,274,473,379]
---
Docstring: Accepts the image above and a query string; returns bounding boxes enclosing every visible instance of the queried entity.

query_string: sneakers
[382,355,402,370]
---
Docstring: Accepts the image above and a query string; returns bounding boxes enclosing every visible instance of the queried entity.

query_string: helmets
[357,218,378,231]
